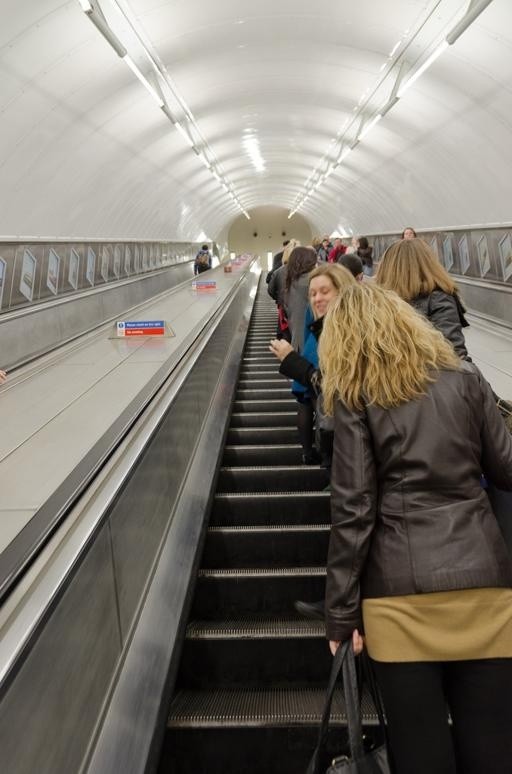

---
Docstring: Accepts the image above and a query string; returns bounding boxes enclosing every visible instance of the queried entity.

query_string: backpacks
[195,251,208,265]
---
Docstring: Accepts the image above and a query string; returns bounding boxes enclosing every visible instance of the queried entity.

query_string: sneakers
[302,454,313,464]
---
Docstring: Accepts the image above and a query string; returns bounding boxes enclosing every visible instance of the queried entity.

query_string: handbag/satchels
[305,638,391,774]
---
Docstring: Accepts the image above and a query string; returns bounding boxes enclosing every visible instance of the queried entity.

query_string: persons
[316,280,511,772]
[193,243,214,274]
[265,227,472,619]
